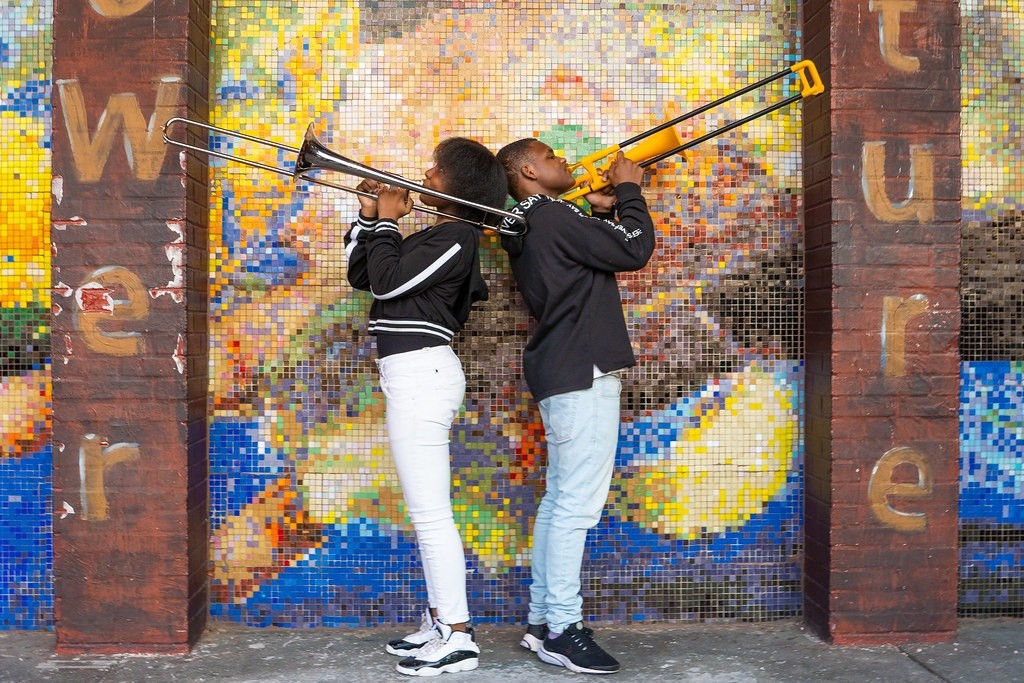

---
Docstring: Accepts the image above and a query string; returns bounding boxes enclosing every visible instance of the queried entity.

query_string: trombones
[161,116,529,238]
[480,59,823,244]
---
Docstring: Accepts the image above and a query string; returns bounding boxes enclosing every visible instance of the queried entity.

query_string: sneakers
[385,607,476,656]
[396,618,480,677]
[520,621,620,673]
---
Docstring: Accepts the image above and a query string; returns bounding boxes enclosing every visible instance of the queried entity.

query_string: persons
[344,137,510,676]
[502,140,654,671]
